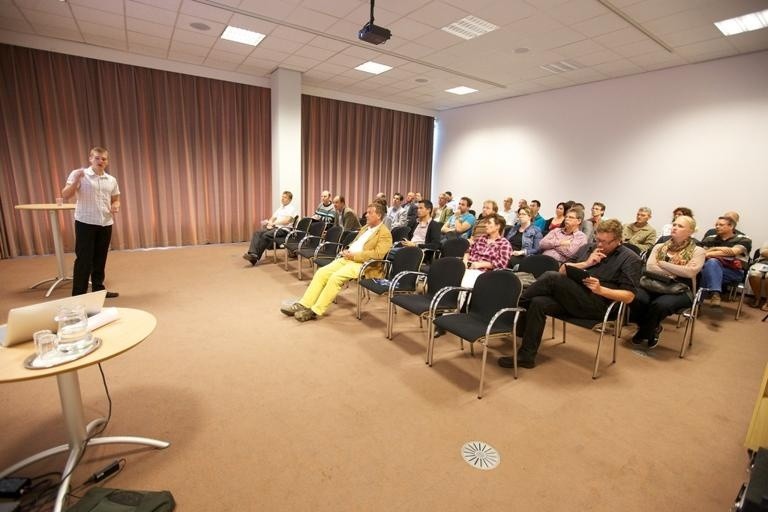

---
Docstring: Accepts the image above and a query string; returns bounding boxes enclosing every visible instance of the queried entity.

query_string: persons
[621,207,751,349]
[361,191,605,338]
[243,191,298,266]
[748,241,767,309]
[61,146,122,297]
[280,203,392,322]
[497,219,642,370]
[288,191,362,259]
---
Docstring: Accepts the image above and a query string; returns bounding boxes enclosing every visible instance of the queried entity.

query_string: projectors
[359,24,391,45]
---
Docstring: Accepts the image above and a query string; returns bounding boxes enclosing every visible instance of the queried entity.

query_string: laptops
[0,290,108,347]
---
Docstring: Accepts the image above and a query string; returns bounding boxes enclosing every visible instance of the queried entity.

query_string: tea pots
[52,306,92,355]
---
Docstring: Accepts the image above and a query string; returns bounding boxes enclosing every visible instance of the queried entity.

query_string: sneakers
[632,325,664,350]
[498,357,535,368]
[281,303,316,322]
[709,293,720,307]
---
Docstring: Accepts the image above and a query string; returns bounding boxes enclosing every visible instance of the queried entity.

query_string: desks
[14,200,82,299]
[0,305,170,512]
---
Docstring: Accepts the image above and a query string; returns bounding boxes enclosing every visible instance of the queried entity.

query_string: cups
[33,329,60,363]
[56,198,64,207]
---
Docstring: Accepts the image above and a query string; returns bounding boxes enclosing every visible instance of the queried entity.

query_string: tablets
[566,264,591,294]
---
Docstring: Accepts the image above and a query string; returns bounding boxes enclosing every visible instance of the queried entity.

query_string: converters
[94,461,119,482]
[0,477,31,497]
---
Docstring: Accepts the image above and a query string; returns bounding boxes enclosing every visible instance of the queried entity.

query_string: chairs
[357,245,424,336]
[625,228,767,360]
[508,252,557,341]
[547,256,626,379]
[388,256,465,365]
[431,238,472,270]
[388,222,413,245]
[428,269,525,399]
[264,216,359,283]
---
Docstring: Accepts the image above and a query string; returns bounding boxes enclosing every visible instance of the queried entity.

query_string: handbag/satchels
[639,275,691,294]
